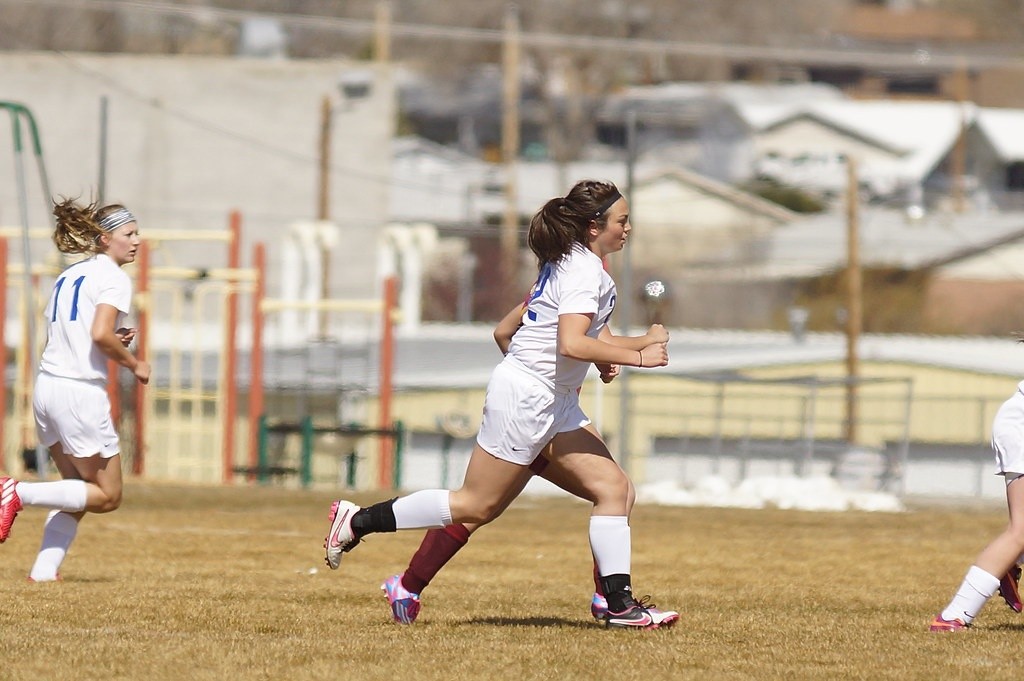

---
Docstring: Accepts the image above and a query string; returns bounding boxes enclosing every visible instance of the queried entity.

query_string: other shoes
[930,613,973,633]
[998,562,1023,614]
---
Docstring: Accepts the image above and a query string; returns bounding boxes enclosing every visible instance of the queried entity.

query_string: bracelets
[638,350,642,367]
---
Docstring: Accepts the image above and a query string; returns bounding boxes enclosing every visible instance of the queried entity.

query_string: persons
[1,195,152,584]
[930,370,1024,630]
[324,179,679,630]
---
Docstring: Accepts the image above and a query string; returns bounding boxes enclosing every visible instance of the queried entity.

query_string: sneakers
[0,477,23,544]
[26,571,62,583]
[605,596,659,631]
[323,500,366,571]
[591,593,680,629]
[381,573,421,625]
